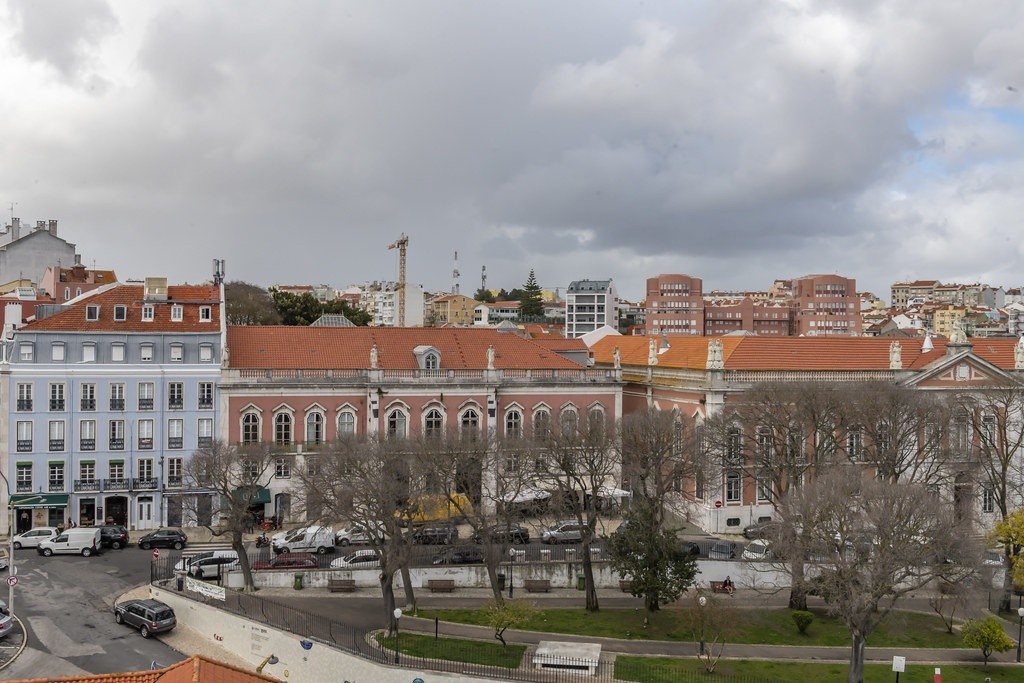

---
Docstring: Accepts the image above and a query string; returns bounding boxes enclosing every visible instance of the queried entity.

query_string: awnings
[8,493,70,509]
[232,489,271,503]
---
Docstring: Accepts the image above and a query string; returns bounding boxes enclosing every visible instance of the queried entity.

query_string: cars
[13,527,60,549]
[174,551,241,581]
[96,526,130,550]
[0,548,10,568]
[472,524,529,545]
[330,550,383,569]
[138,527,188,550]
[115,599,176,639]
[0,599,13,637]
[432,546,485,564]
[271,528,299,545]
[541,521,935,560]
[400,524,458,545]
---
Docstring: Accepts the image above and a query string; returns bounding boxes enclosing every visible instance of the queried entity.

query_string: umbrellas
[496,485,552,522]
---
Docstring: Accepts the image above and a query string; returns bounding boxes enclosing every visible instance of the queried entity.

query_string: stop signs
[715,501,722,507]
[153,548,159,558]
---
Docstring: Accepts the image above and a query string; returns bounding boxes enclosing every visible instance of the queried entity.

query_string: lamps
[257,654,278,674]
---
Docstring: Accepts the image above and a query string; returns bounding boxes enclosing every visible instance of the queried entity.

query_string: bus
[395,494,474,527]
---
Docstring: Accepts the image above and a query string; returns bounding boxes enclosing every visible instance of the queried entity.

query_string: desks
[533,640,601,675]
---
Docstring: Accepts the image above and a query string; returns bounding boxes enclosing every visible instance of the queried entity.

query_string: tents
[585,486,630,508]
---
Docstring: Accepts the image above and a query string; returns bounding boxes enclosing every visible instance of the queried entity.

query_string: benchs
[524,580,552,592]
[327,580,356,592]
[710,581,736,593]
[428,580,455,593]
[620,580,633,592]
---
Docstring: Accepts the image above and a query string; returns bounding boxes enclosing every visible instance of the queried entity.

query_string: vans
[273,526,335,555]
[335,519,385,546]
[37,528,101,557]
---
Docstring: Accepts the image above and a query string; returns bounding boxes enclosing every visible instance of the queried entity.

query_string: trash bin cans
[498,573,505,590]
[577,574,585,591]
[294,574,303,590]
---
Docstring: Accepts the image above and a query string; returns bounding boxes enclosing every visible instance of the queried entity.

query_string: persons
[21,510,29,532]
[271,514,277,527]
[723,576,733,597]
[67,518,77,528]
[242,510,264,533]
[690,543,700,554]
